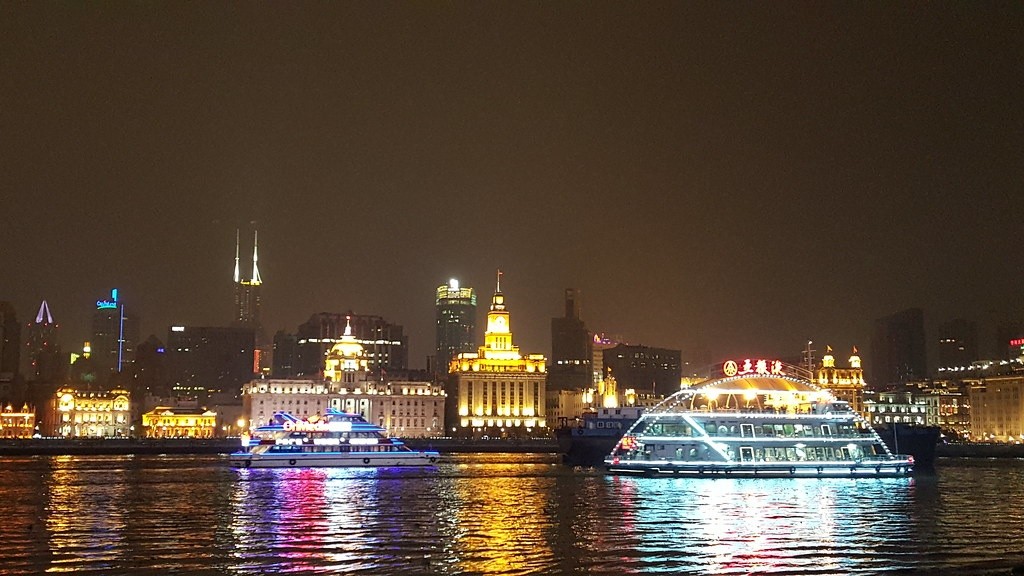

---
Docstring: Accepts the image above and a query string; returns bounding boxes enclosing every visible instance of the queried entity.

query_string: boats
[216,413,441,467]
[604,358,920,476]
